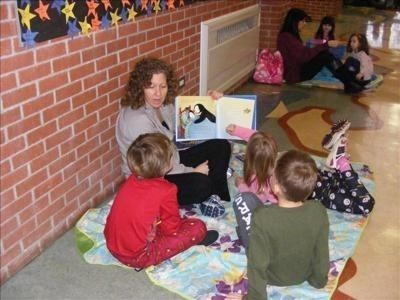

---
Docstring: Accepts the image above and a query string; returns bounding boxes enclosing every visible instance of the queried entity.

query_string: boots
[326,55,372,93]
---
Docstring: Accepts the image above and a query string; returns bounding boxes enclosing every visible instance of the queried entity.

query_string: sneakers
[321,118,352,168]
[198,230,219,248]
[199,193,225,217]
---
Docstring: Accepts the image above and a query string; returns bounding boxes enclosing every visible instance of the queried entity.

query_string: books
[175,95,257,141]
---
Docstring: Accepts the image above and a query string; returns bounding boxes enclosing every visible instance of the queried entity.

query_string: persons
[115,58,231,218]
[276,7,377,94]
[224,119,375,300]
[104,133,219,268]
